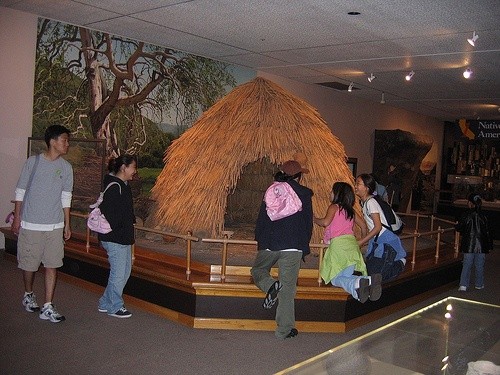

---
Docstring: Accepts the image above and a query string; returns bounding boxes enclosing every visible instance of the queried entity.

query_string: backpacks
[366,195,397,231]
[263,181,303,221]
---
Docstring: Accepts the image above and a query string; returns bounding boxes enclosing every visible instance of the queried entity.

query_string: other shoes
[263,281,284,309]
[400,258,406,265]
[476,285,484,289]
[358,278,369,304]
[283,328,299,338]
[369,273,382,301]
[458,285,466,292]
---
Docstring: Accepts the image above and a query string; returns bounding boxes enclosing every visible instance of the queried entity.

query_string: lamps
[367,72,375,83]
[348,82,355,92]
[405,69,416,81]
[467,30,480,47]
[379,92,386,105]
[462,67,474,79]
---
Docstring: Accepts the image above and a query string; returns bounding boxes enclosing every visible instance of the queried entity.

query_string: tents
[153,77,370,258]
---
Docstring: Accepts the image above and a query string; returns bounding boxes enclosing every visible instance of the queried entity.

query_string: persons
[97,153,137,319]
[312,182,382,304]
[13,125,73,323]
[250,161,313,340]
[357,162,403,282]
[454,194,493,292]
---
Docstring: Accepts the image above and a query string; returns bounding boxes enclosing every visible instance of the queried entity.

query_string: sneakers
[108,305,133,318]
[39,304,66,323]
[22,292,40,313]
[98,305,108,312]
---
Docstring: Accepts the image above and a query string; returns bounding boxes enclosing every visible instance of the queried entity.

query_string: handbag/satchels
[6,210,20,236]
[388,207,403,231]
[87,182,121,234]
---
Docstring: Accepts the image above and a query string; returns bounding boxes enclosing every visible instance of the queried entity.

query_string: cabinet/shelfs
[372,129,438,216]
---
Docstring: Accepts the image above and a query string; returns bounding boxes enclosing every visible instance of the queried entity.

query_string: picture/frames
[27,135,107,210]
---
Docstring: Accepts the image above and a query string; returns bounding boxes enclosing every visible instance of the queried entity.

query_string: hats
[277,160,310,176]
